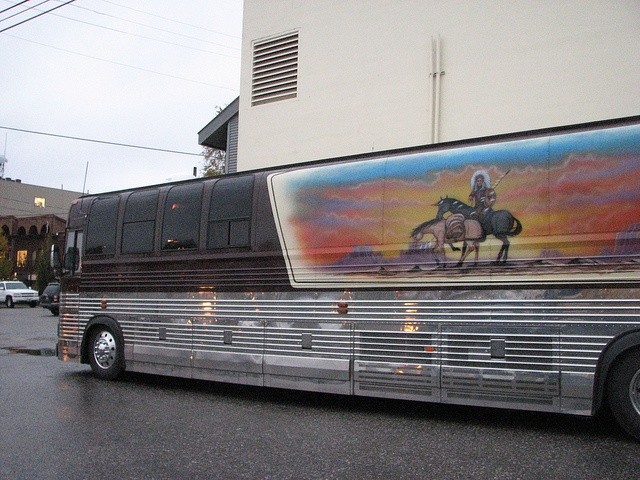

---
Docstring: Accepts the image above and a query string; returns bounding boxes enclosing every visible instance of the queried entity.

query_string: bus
[56,114,640,442]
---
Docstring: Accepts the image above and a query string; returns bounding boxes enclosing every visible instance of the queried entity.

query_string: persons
[468,175,497,235]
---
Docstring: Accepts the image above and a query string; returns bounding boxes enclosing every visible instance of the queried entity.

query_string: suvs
[0,280,39,308]
[40,283,61,316]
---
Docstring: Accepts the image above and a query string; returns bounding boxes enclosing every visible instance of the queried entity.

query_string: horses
[431,194,522,267]
[410,217,486,268]
